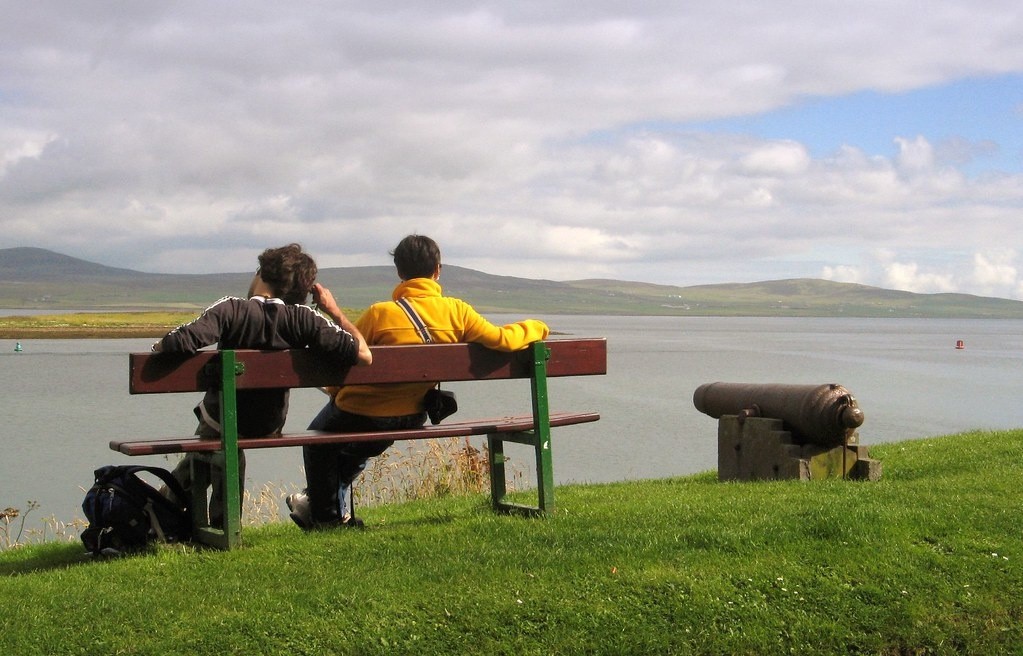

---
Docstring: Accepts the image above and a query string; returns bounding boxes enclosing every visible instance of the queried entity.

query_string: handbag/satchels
[426,386,457,425]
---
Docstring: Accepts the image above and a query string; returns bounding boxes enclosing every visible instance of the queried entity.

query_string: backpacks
[80,464,193,561]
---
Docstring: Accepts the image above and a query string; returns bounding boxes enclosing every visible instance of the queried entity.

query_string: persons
[283,233,551,531]
[151,242,373,533]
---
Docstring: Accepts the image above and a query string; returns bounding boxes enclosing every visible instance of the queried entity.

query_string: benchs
[109,337,606,552]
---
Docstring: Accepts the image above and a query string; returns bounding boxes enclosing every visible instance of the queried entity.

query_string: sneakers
[287,490,351,530]
